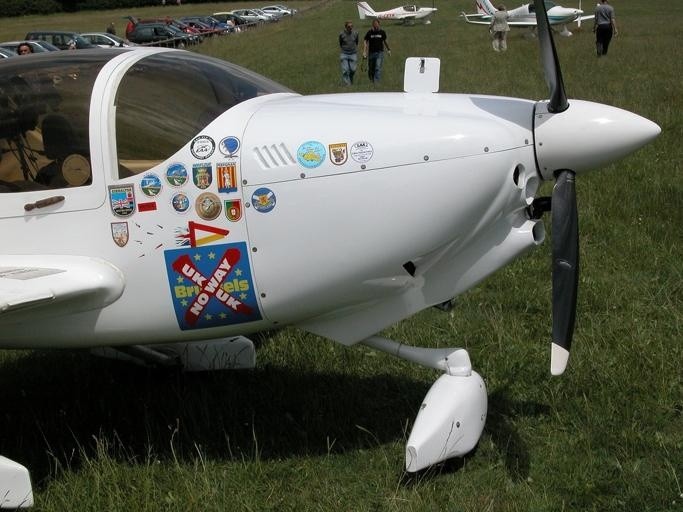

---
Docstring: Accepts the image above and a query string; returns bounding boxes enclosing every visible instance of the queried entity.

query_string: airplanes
[458,0,597,36]
[0,0,661,512]
[353,0,438,27]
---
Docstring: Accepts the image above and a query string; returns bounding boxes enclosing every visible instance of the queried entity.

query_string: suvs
[120,15,198,49]
[124,16,204,46]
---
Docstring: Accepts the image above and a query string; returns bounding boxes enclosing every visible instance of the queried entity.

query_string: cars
[1,39,81,84]
[180,3,298,37]
[0,49,43,84]
[68,33,139,52]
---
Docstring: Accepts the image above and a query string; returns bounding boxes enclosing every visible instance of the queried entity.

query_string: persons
[489,3,510,52]
[107,23,116,35]
[362,20,391,83]
[339,20,359,86]
[17,43,49,115]
[592,0,618,57]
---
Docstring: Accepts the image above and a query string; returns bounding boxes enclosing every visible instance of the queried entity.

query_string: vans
[22,30,102,52]
